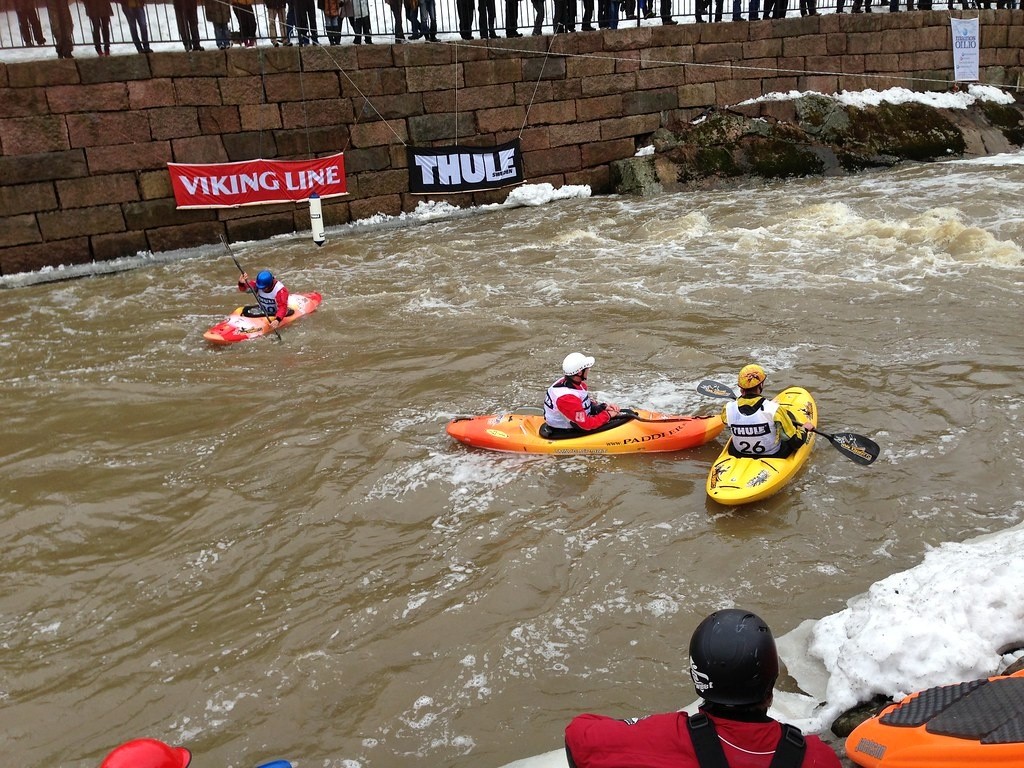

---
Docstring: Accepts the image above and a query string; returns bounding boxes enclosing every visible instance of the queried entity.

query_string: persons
[239,271,289,330]
[47,0,74,59]
[174,0,204,51]
[324,0,374,46]
[121,0,153,54]
[457,0,501,40]
[721,364,815,455]
[566,609,842,768]
[277,0,321,47]
[385,0,441,44]
[206,0,231,49]
[543,353,621,433]
[83,0,114,58]
[101,739,192,768]
[553,0,788,34]
[16,0,45,46]
[264,0,293,47]
[232,0,256,48]
[505,0,544,38]
[800,0,1024,18]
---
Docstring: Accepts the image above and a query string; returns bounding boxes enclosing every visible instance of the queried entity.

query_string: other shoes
[217,43,231,50]
[37,38,45,45]
[244,40,254,47]
[135,43,154,53]
[98,48,111,57]
[183,43,205,51]
[57,50,74,59]
[270,10,873,45]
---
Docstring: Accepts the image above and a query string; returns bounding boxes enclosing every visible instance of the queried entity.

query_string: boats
[704,385,818,506]
[203,291,323,346]
[444,405,727,457]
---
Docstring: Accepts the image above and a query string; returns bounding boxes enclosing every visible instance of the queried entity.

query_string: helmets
[256,270,272,289]
[738,365,767,390]
[689,610,777,704]
[562,352,595,375]
[100,738,192,768]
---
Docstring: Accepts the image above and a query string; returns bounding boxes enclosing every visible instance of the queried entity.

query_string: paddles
[616,412,693,424]
[697,379,881,466]
[218,233,283,341]
